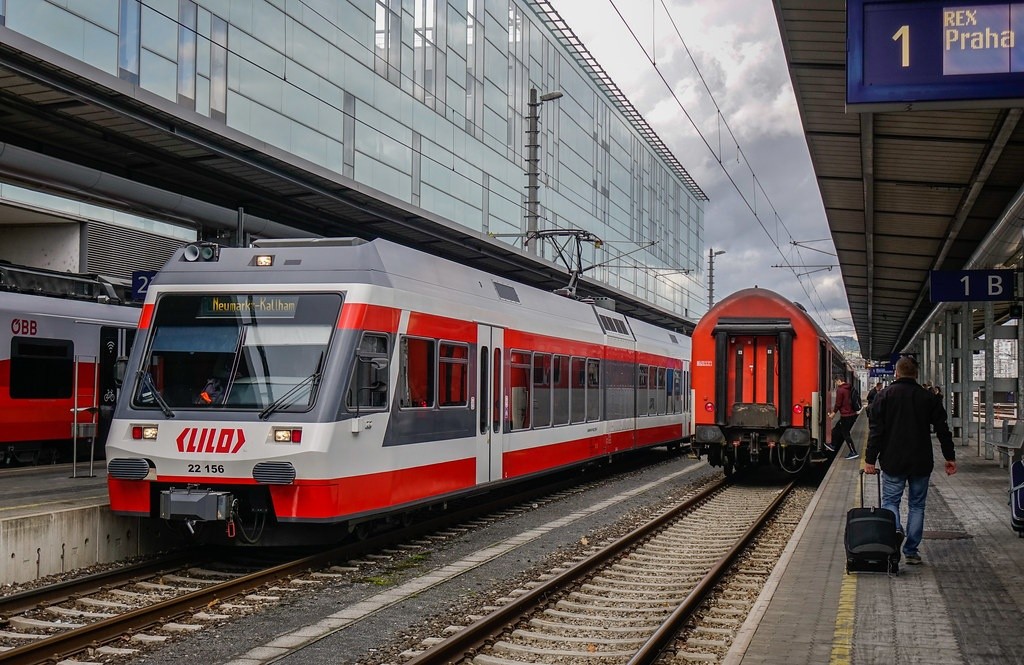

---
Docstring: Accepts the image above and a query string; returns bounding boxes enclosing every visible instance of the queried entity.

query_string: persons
[862,356,958,566]
[823,373,861,460]
[865,378,946,435]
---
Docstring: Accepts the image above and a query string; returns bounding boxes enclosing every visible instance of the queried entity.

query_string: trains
[689,284,864,482]
[1,259,145,468]
[103,228,696,551]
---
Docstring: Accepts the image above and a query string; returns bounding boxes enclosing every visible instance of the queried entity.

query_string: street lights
[707,247,726,312]
[524,85,564,254]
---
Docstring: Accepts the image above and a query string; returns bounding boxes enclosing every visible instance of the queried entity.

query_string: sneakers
[905,553,921,565]
[824,442,835,451]
[845,452,859,459]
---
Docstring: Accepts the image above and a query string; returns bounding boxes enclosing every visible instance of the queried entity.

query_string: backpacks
[851,387,862,411]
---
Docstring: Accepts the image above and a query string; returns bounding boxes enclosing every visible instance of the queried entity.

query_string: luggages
[1009,460,1024,537]
[846,468,899,576]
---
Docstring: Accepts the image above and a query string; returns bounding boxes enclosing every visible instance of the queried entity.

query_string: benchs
[982,418,1024,475]
